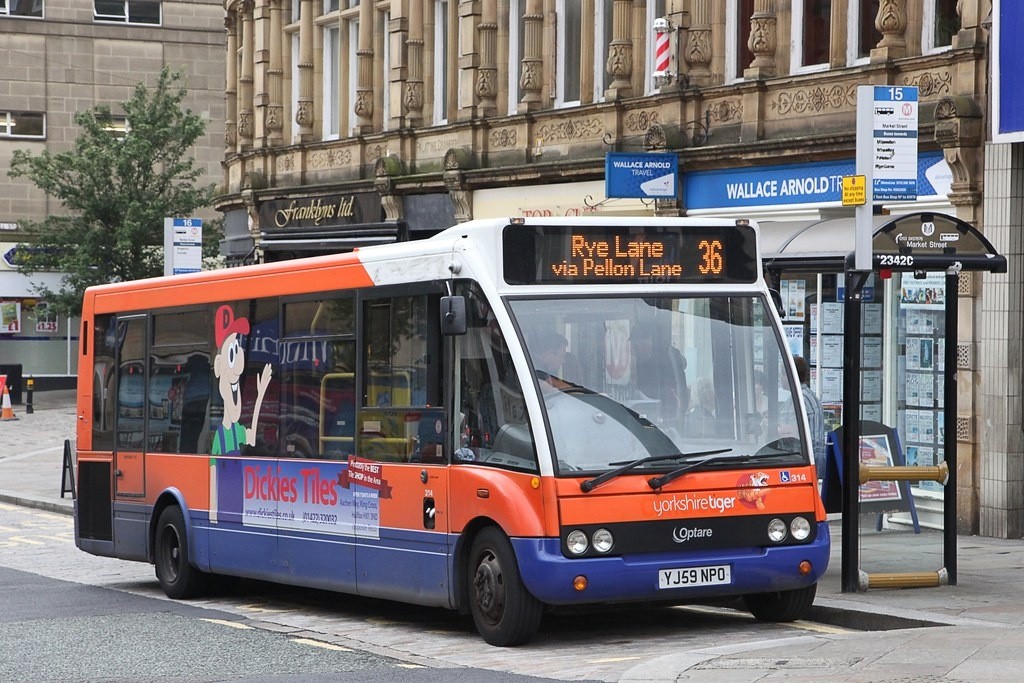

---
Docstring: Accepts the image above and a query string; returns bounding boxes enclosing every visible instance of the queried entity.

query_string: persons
[752,370,770,444]
[688,381,724,437]
[628,324,689,431]
[532,333,585,398]
[775,355,828,481]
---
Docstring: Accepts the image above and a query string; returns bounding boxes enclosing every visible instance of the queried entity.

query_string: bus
[71,208,831,646]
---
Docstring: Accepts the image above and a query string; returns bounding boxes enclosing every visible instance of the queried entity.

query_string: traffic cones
[1,385,19,420]
